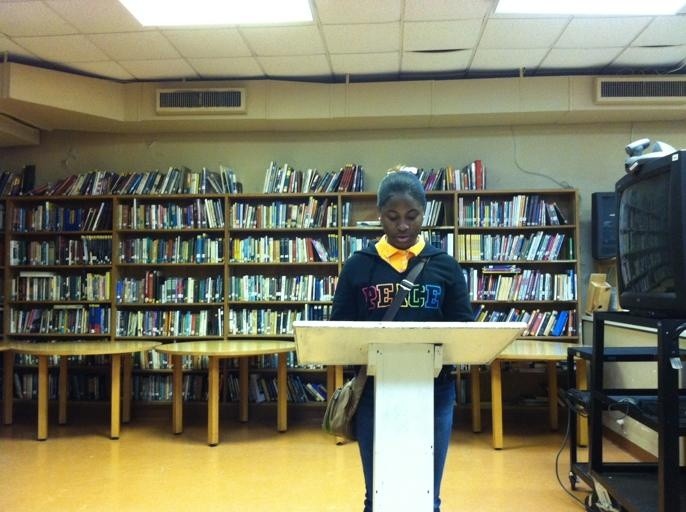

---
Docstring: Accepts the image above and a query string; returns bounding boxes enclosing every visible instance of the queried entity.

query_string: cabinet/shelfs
[0,188,585,422]
[587,310,685,512]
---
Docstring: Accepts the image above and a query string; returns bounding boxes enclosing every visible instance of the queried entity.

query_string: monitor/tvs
[591,191,618,260]
[613,150,686,320]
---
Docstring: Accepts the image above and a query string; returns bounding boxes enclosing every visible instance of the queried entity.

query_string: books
[342,202,349,227]
[116,199,225,402]
[11,203,113,408]
[456,190,581,347]
[342,234,372,268]
[391,161,486,192]
[420,200,456,259]
[340,162,364,194]
[230,200,339,403]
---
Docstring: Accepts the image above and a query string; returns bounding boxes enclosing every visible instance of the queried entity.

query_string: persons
[0,162,342,195]
[328,170,473,512]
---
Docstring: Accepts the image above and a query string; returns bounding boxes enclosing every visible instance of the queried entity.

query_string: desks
[0,341,28,425]
[154,340,296,446]
[10,341,162,440]
[470,343,594,449]
[327,365,345,446]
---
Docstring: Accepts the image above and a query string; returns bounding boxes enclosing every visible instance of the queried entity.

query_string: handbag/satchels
[322,375,366,442]
